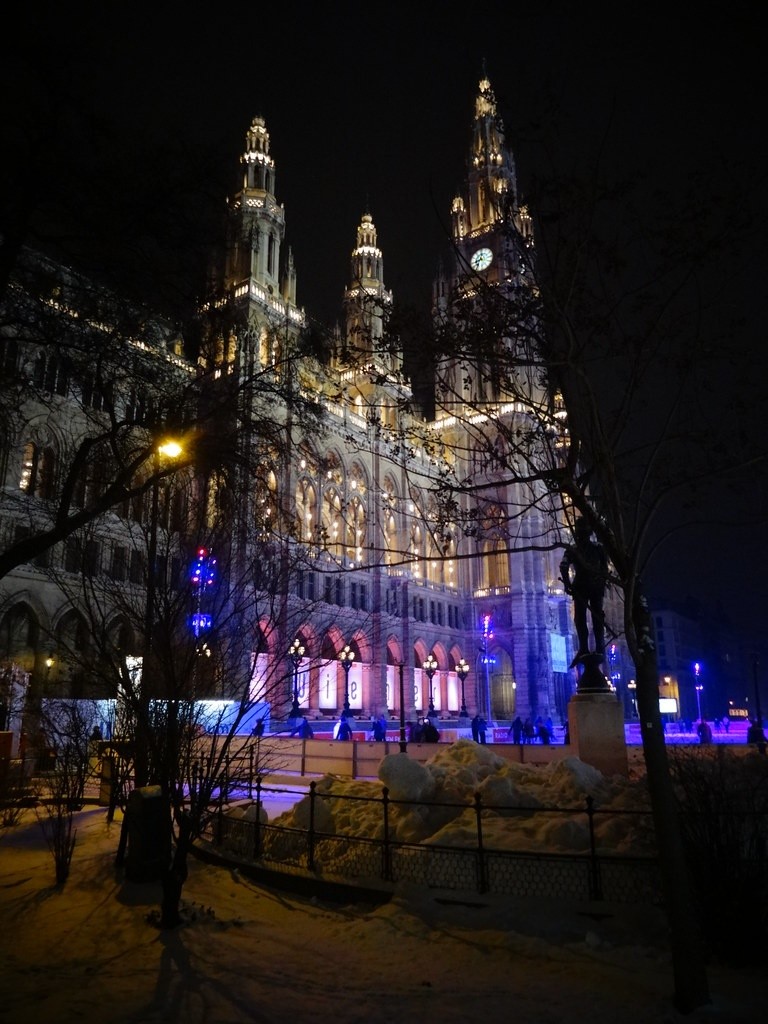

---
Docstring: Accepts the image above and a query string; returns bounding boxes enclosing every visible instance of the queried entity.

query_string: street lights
[423,655,439,718]
[287,638,304,727]
[136,441,180,787]
[627,679,639,719]
[455,659,470,718]
[44,656,54,695]
[339,645,355,718]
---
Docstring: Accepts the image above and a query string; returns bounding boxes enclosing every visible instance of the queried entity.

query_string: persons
[506,716,570,745]
[89,726,102,741]
[470,715,488,744]
[251,718,265,736]
[334,716,352,741]
[288,717,314,739]
[560,516,609,670]
[660,716,730,734]
[697,718,713,744]
[369,714,441,743]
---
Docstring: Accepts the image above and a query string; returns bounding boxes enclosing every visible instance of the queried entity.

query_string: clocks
[470,247,493,272]
